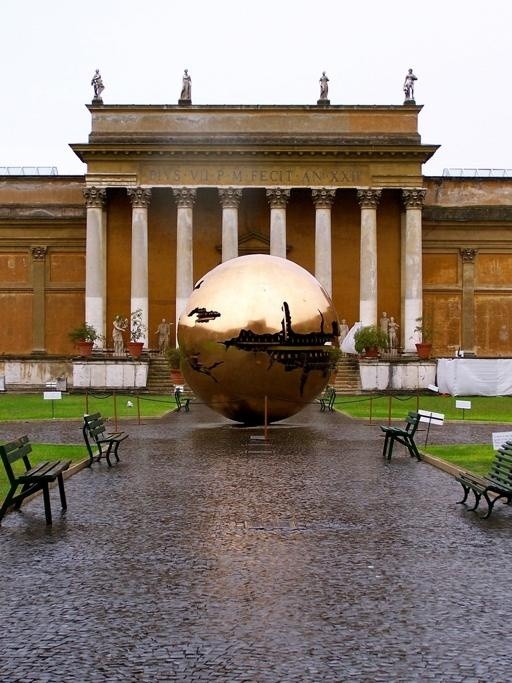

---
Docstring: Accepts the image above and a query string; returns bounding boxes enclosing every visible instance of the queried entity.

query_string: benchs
[321,386,335,413]
[1,412,130,526]
[380,411,512,519]
[175,389,193,412]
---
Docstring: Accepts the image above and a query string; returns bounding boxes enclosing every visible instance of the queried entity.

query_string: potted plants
[123,308,147,356]
[68,320,99,356]
[354,325,389,358]
[168,347,185,386]
[415,317,435,358]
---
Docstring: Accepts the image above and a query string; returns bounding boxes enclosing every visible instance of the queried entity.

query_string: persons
[380,310,389,334]
[91,68,105,96]
[155,318,171,349]
[181,68,192,100]
[112,314,128,351]
[403,67,418,98]
[388,315,400,349]
[337,317,350,343]
[319,71,330,97]
